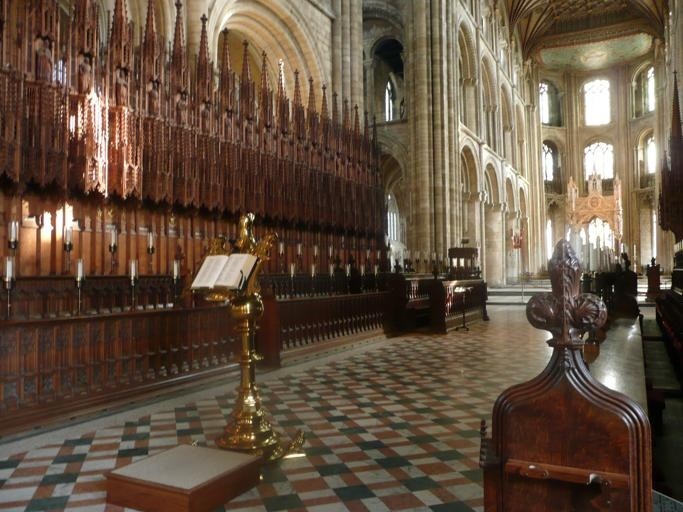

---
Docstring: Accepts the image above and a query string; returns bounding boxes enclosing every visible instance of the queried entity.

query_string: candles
[314,245,318,257]
[78,262,83,279]
[149,234,153,249]
[313,266,314,277]
[10,221,15,241]
[66,229,70,244]
[175,263,178,278]
[7,260,12,278]
[299,245,301,255]
[280,243,284,254]
[330,247,447,274]
[291,266,294,277]
[130,264,136,277]
[111,229,115,245]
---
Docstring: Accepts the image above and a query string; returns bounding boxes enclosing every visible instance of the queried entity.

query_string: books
[191,254,257,290]
